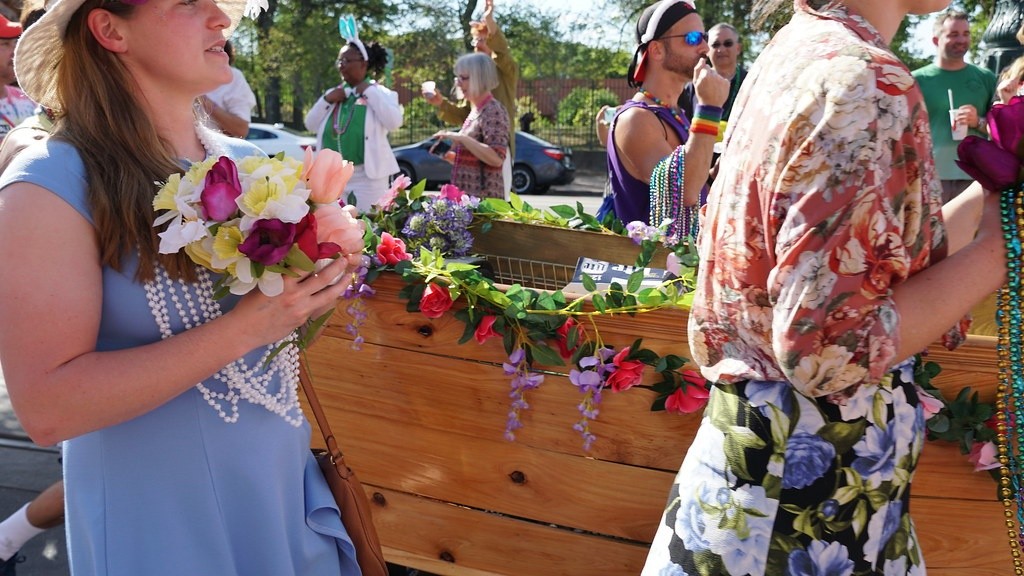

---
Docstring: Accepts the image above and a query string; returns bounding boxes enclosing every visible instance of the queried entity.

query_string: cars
[389,127,578,195]
[245,122,318,163]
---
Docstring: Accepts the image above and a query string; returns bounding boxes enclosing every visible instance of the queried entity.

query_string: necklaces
[335,82,356,158]
[136,122,304,429]
[649,145,703,242]
[995,187,1024,576]
[638,85,693,132]
[452,97,495,186]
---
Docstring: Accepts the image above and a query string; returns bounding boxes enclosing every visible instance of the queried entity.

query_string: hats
[633,0,698,81]
[0,14,22,38]
[13,0,245,111]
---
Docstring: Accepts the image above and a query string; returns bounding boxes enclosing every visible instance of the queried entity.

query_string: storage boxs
[560,255,689,306]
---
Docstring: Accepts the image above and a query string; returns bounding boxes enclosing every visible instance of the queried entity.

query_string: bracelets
[688,105,722,135]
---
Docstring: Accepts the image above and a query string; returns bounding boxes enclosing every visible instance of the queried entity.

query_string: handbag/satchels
[310,448,389,576]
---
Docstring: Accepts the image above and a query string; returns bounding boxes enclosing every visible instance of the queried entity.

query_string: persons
[193,40,258,138]
[643,0,1024,576]
[432,52,512,200]
[1,0,70,576]
[0,0,367,576]
[421,0,520,212]
[910,10,998,208]
[678,24,748,181]
[303,17,404,217]
[0,13,38,142]
[593,0,731,242]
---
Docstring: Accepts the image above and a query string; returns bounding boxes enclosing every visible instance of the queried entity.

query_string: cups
[949,109,967,140]
[420,81,436,98]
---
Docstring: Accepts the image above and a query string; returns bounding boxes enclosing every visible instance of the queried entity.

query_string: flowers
[346,174,1017,503]
[951,94,1024,193]
[149,145,370,384]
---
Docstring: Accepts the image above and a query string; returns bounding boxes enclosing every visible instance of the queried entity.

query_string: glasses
[456,76,469,83]
[336,58,362,66]
[651,31,709,46]
[711,41,739,49]
